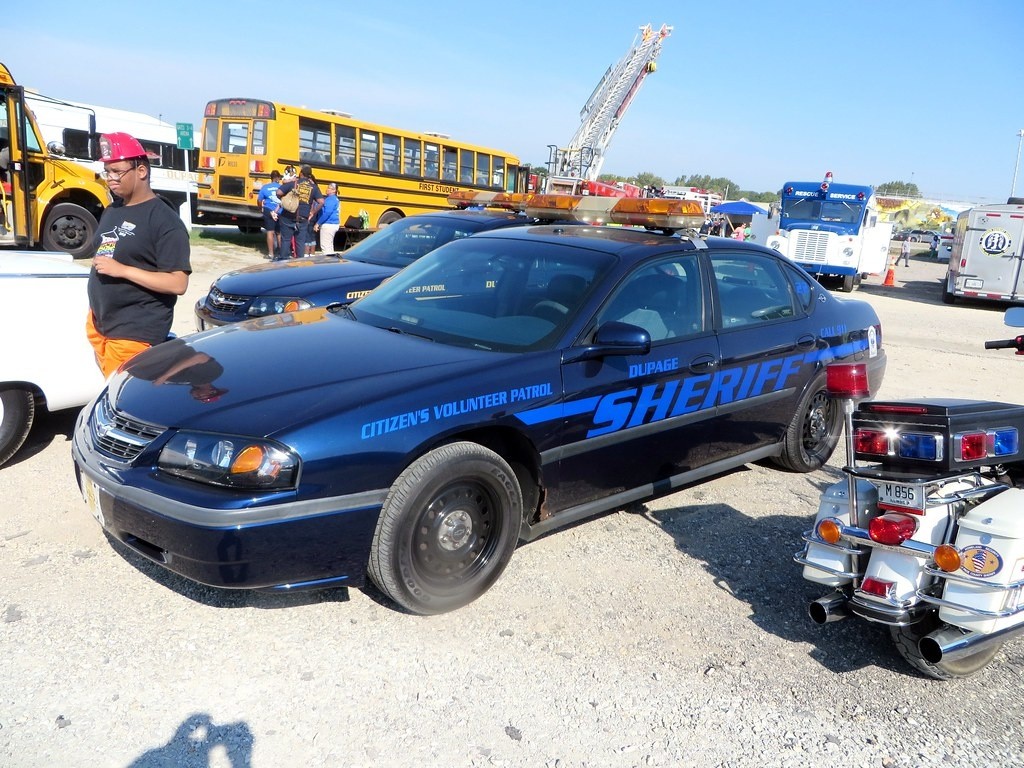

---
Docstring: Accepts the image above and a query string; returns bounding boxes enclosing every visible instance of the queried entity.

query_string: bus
[0,88,202,226]
[0,63,114,260]
[193,99,529,236]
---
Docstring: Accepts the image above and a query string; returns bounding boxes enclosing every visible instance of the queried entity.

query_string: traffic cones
[881,255,896,286]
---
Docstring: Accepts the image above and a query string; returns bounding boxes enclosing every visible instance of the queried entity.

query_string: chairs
[343,227,357,251]
[608,274,686,341]
[538,273,587,324]
[233,146,503,188]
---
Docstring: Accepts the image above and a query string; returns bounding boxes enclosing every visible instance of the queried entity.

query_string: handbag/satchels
[281,177,299,213]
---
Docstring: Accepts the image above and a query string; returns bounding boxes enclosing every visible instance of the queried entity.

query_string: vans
[750,171,894,294]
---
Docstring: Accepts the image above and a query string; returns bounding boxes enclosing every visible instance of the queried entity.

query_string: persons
[895,237,911,267]
[257,164,341,261]
[86,133,192,381]
[425,151,436,170]
[700,213,752,243]
[118,340,230,404]
[928,235,938,261]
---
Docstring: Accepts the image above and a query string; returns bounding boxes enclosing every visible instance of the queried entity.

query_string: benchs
[717,280,790,323]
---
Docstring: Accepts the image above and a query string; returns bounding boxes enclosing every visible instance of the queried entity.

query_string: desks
[344,228,435,256]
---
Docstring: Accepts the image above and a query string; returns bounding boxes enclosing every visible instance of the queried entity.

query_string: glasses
[101,164,144,182]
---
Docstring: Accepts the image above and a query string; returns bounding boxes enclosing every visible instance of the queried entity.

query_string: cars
[910,229,941,243]
[892,231,908,242]
[194,193,593,333]
[0,245,176,471]
[71,193,887,618]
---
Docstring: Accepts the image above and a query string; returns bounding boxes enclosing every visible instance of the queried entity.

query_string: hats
[300,163,313,178]
[98,132,148,163]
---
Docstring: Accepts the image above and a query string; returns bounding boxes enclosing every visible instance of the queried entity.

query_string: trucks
[942,200,1024,309]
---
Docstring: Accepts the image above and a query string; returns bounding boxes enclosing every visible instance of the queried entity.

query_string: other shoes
[263,253,272,260]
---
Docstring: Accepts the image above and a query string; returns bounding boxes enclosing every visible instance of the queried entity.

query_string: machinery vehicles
[543,21,675,228]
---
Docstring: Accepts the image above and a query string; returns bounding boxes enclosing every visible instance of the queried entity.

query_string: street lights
[1010,129,1024,197]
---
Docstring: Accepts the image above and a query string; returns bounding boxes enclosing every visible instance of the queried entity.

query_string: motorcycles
[792,306,1024,680]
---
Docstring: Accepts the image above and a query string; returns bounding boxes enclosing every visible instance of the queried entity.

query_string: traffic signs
[176,122,194,150]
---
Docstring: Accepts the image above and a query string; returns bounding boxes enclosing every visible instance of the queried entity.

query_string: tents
[711,201,770,216]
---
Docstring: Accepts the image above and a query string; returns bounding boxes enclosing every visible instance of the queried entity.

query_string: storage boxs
[939,489,1024,633]
[853,397,1024,470]
[803,479,883,586]
